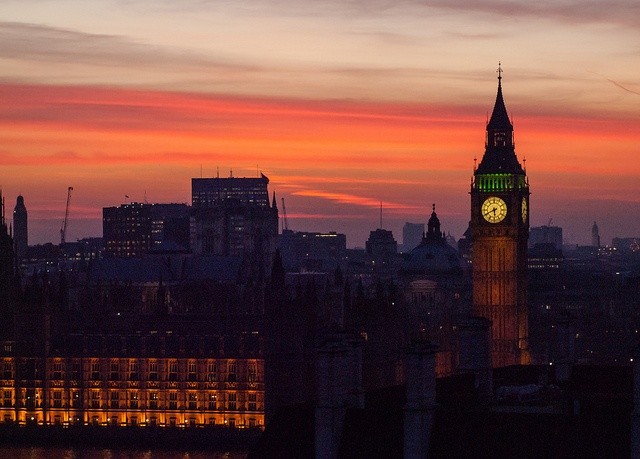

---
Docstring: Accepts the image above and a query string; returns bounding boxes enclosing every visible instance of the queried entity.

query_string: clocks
[521,197,527,224]
[481,197,507,224]
